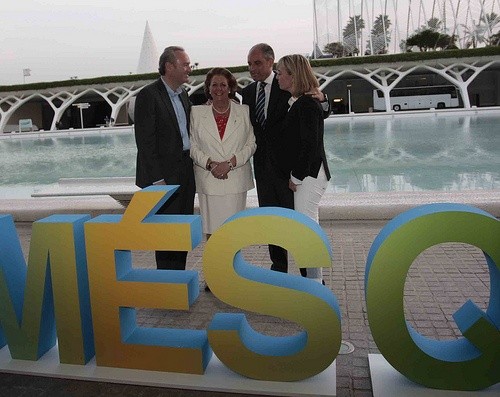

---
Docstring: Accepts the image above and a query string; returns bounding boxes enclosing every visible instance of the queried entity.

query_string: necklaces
[213,106,229,114]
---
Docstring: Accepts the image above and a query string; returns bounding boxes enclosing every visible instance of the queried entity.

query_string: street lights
[347,84,352,114]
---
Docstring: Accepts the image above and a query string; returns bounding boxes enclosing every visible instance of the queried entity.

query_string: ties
[256,81,265,129]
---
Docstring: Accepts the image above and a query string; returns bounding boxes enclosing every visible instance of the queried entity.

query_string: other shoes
[204,281,210,292]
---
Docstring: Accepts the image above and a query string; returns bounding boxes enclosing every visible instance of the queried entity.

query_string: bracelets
[211,165,217,171]
[227,160,234,169]
[207,161,213,170]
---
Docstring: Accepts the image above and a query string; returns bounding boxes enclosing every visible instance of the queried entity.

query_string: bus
[373,85,460,112]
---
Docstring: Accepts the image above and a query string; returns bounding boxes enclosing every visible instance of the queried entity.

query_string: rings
[221,174,223,176]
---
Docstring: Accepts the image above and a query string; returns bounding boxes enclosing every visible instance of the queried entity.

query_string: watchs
[320,94,328,102]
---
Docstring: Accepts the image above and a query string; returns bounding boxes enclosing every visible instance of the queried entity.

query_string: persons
[206,43,330,285]
[275,54,331,284]
[190,66,257,292]
[135,46,196,270]
